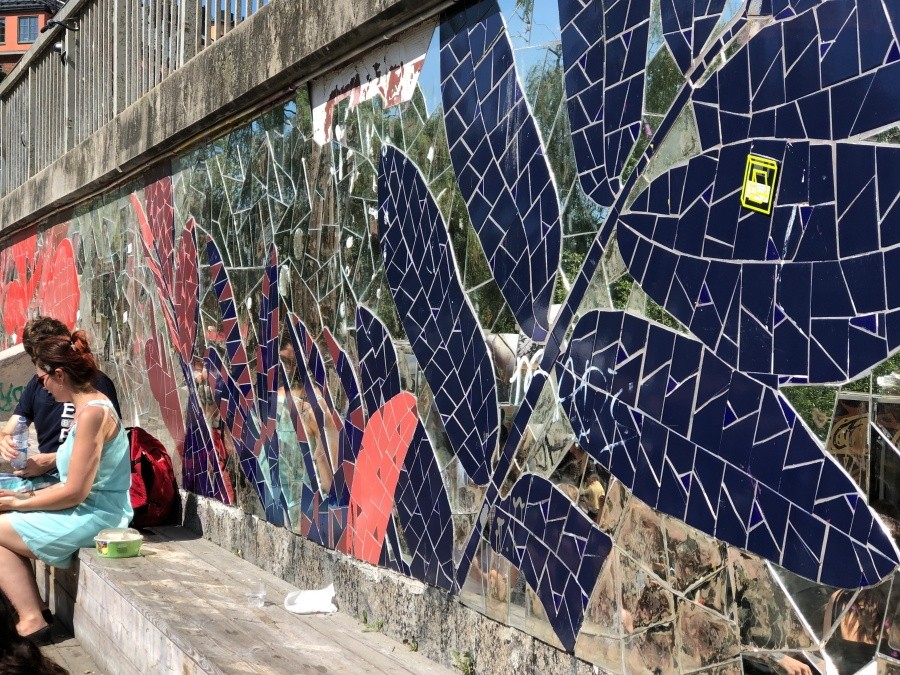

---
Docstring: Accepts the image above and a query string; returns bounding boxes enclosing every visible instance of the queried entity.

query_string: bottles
[11,417,29,469]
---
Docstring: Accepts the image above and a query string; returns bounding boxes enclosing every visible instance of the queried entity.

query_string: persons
[255,345,337,538]
[0,332,137,646]
[0,317,123,491]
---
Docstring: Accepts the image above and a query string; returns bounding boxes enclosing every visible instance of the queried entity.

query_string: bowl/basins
[94,528,144,558]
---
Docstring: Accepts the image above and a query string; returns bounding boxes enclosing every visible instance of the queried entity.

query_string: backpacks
[123,426,184,528]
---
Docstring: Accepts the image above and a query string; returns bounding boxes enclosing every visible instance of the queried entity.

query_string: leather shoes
[12,608,54,628]
[22,623,53,647]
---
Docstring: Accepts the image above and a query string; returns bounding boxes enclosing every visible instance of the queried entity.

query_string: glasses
[36,373,49,387]
